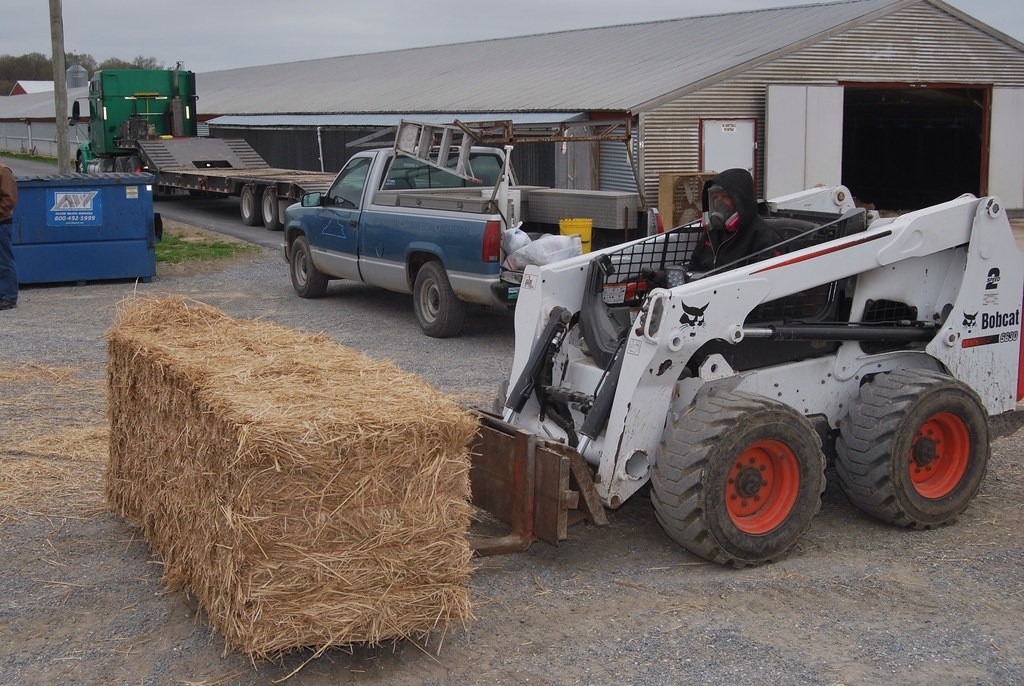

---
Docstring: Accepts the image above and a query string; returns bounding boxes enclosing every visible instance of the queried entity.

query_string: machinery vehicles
[467,184,1024,571]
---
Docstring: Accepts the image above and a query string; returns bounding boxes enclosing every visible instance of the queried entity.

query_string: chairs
[764,218,821,254]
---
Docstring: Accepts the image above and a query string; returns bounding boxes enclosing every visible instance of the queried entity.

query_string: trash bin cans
[10,173,156,283]
[560,217,593,254]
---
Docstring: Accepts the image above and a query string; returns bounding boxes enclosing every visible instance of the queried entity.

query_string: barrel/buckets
[559,217,595,255]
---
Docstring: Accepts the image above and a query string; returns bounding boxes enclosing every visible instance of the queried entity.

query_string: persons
[0,163,19,309]
[682,168,787,321]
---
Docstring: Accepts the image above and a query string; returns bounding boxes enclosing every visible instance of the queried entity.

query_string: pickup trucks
[283,144,665,339]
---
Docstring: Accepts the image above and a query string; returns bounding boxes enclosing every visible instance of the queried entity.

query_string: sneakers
[0,300,17,310]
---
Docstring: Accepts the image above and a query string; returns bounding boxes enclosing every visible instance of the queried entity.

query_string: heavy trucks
[68,60,397,232]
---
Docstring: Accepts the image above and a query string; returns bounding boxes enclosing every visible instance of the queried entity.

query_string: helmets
[708,184,736,231]
